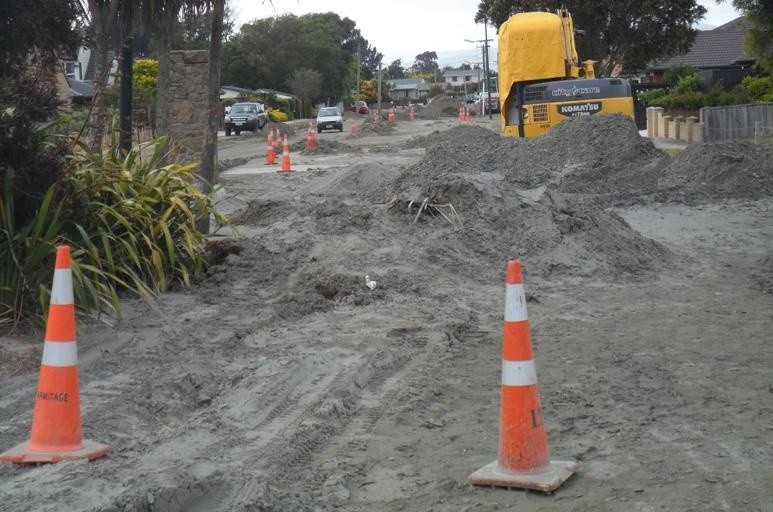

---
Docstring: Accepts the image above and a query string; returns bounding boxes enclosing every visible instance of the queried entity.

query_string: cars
[348,100,369,114]
[224,101,268,137]
[317,106,344,132]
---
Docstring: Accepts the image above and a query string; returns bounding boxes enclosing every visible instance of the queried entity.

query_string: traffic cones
[350,119,358,133]
[386,108,397,121]
[306,121,319,148]
[409,107,415,120]
[458,106,470,122]
[265,126,296,172]
[0,246,110,462]
[466,259,580,494]
[372,112,379,122]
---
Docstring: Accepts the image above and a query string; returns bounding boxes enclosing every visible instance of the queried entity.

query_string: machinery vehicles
[495,9,635,138]
